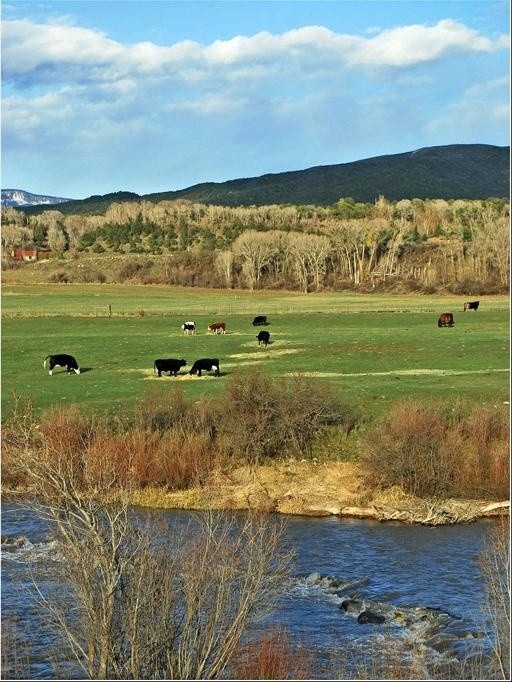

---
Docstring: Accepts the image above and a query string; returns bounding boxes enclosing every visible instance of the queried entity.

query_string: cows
[43,353,80,376]
[189,358,220,378]
[438,312,454,327]
[464,301,479,312]
[207,322,225,335]
[154,358,187,377]
[256,330,270,346]
[252,316,267,327]
[181,321,196,335]
